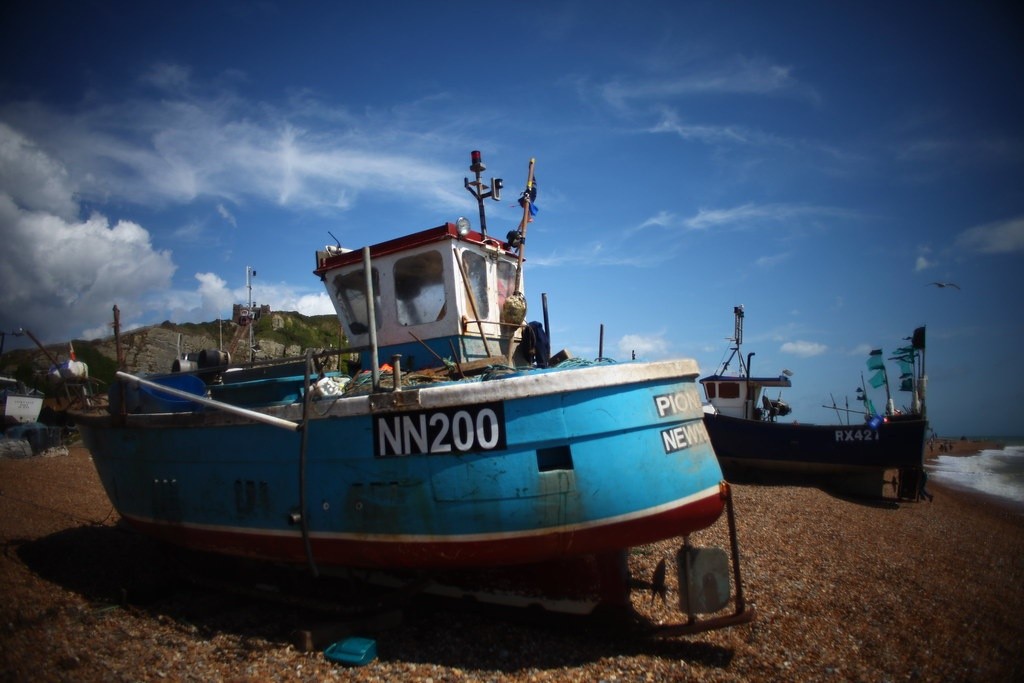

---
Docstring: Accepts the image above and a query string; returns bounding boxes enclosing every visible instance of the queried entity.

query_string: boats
[56,147,761,642]
[699,301,931,503]
